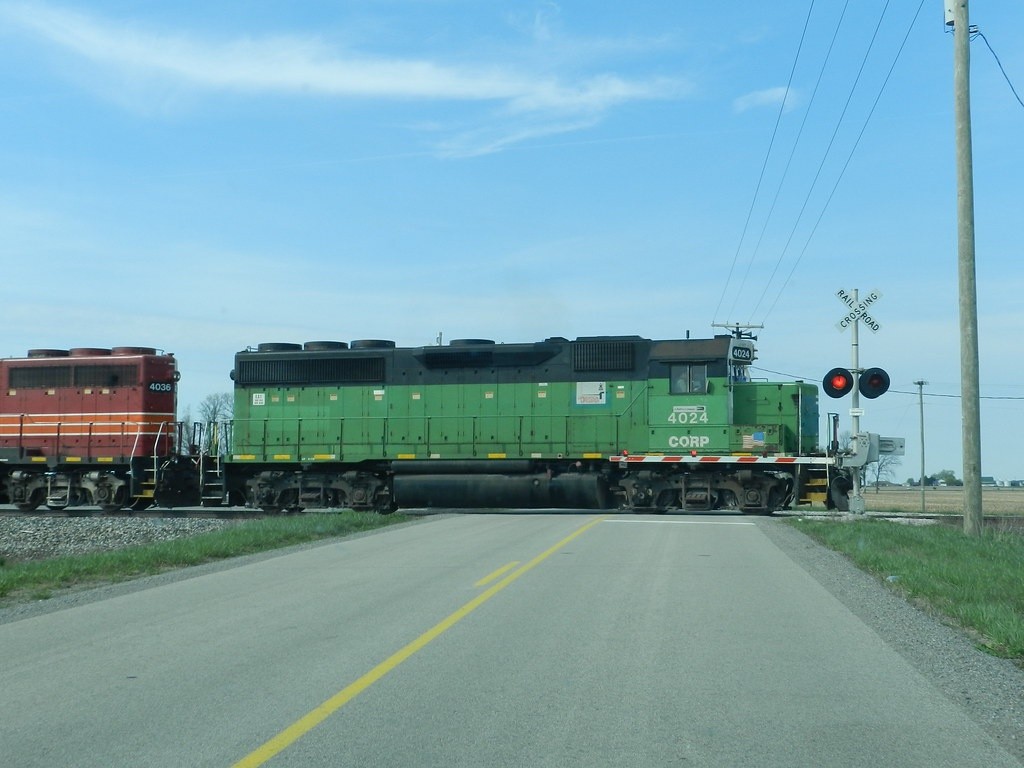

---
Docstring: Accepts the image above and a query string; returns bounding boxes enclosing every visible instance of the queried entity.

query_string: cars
[981,476,1024,488]
[901,481,919,487]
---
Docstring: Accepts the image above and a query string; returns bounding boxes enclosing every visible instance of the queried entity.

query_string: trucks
[933,479,946,487]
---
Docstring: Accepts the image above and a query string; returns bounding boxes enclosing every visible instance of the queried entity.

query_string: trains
[0,337,855,516]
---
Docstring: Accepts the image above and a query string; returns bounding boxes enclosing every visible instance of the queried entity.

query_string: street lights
[914,379,929,514]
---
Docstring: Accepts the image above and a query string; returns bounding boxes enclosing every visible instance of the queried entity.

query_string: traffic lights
[861,367,890,399]
[828,368,853,397]
